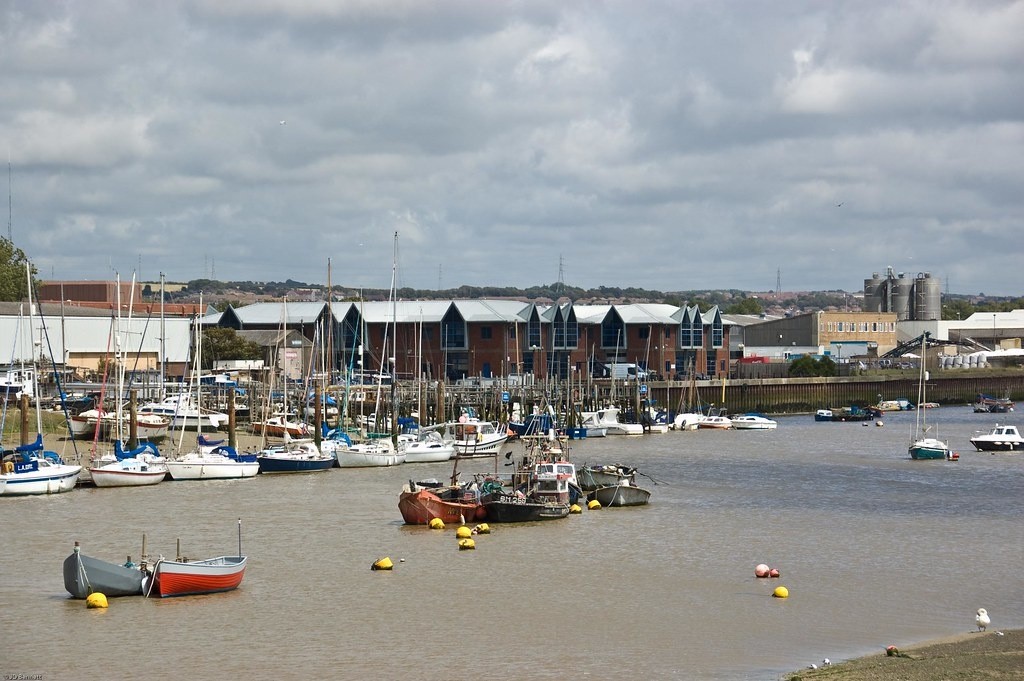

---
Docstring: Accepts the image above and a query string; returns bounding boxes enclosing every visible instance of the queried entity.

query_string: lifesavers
[477,433,483,442]
[4,461,15,473]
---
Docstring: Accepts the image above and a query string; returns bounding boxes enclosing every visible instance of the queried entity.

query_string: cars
[673,370,710,381]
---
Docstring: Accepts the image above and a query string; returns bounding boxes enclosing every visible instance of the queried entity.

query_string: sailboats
[908,330,951,461]
[0,230,1016,527]
[0,260,83,497]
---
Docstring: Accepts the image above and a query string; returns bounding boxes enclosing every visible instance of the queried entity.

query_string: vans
[602,364,648,382]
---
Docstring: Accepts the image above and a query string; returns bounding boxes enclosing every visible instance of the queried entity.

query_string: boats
[63,531,153,600]
[969,422,1024,452]
[151,517,247,598]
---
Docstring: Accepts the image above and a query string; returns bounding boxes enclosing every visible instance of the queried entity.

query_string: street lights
[737,344,744,379]
[836,344,843,377]
